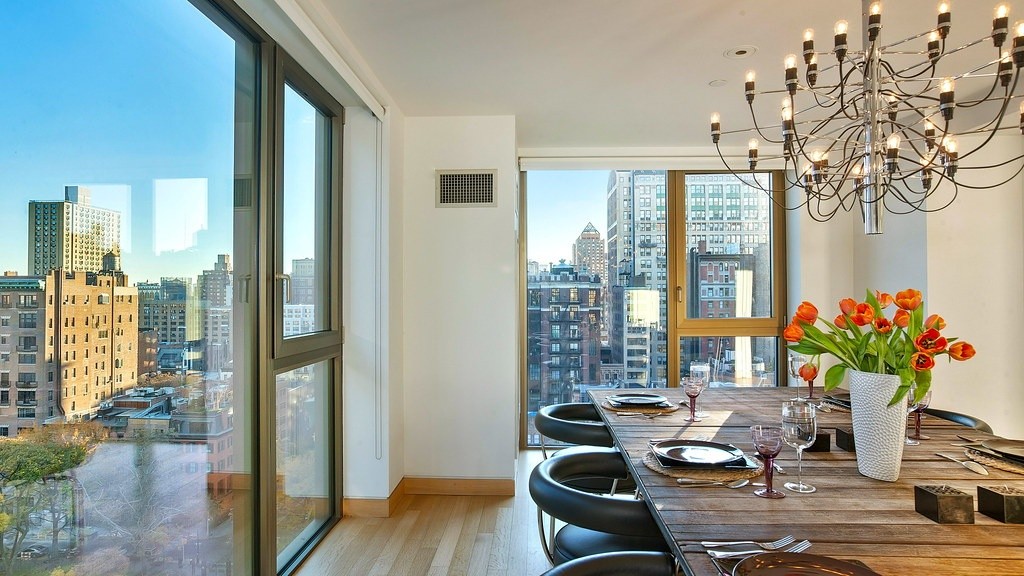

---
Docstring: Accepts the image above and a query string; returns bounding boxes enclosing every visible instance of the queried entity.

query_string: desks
[585,386,1024,576]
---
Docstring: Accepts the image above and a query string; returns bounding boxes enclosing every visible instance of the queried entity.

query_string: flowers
[783,288,976,408]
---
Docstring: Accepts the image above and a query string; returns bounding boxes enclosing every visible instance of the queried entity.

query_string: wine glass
[750,425,786,498]
[800,355,820,399]
[783,401,816,493]
[788,356,806,401]
[683,377,704,422]
[909,391,932,440]
[904,388,920,445]
[690,361,710,418]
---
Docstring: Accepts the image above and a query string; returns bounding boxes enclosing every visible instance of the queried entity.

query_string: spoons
[805,401,831,412]
[679,400,698,412]
[680,479,749,488]
[754,451,785,473]
[936,453,989,476]
[617,411,661,418]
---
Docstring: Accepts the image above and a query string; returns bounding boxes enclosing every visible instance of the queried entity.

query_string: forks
[707,539,812,559]
[701,534,796,550]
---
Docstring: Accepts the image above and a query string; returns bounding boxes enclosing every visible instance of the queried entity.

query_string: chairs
[922,408,993,436]
[529,449,663,567]
[540,551,686,576]
[536,402,620,460]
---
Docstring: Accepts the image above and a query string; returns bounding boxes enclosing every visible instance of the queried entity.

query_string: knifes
[677,478,767,486]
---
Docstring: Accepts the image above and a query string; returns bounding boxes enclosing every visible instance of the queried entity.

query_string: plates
[610,393,667,404]
[821,396,851,408]
[832,393,851,401]
[710,558,874,576]
[981,439,1024,458]
[653,440,743,465]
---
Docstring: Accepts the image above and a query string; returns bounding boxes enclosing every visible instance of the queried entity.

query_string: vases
[848,369,909,482]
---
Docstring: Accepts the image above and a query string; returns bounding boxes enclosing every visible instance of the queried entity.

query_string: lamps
[710,0,1024,235]
